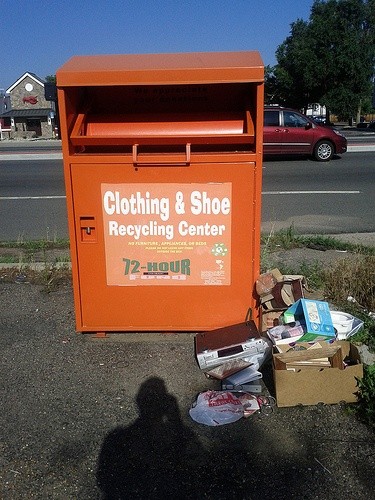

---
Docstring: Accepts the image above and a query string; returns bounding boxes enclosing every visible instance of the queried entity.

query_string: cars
[263,103,348,162]
[306,114,327,127]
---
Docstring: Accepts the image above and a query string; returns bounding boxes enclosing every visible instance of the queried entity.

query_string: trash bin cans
[56,50,264,332]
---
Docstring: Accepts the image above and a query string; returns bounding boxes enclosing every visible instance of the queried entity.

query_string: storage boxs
[255,268,364,408]
